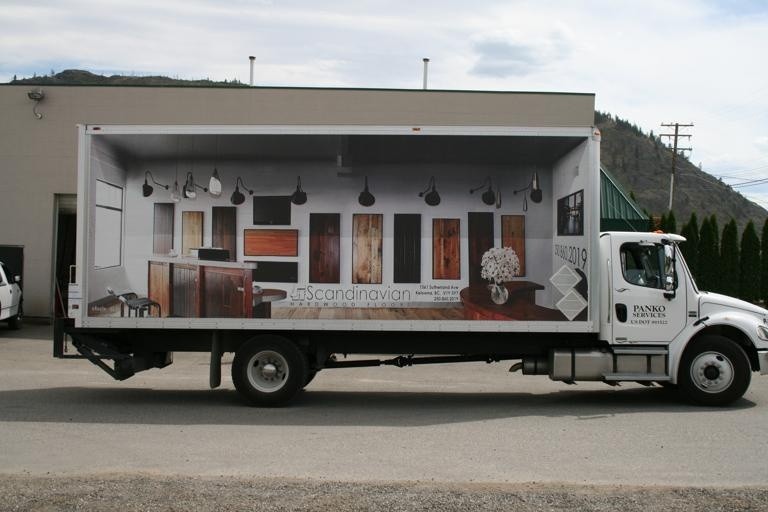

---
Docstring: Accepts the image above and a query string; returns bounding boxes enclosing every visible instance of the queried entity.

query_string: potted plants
[481,246,521,304]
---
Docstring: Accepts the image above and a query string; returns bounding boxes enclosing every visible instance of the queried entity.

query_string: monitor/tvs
[252,195,292,225]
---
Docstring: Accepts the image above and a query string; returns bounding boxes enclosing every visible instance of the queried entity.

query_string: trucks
[50,113,768,411]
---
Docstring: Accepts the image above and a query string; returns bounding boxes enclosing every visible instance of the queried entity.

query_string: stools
[107,286,137,317]
[119,297,162,317]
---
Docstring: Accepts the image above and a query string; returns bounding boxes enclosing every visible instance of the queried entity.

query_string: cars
[0,259,26,331]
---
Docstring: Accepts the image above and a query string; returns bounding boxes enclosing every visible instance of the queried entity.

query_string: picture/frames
[556,189,584,236]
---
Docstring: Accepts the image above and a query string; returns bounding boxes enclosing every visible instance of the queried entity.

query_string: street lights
[247,55,257,87]
[421,57,430,90]
